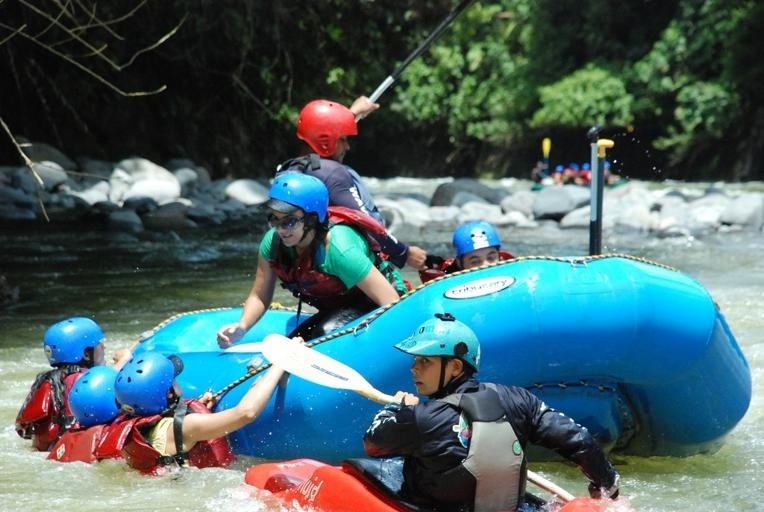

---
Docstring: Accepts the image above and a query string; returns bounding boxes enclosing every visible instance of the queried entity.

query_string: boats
[531,179,631,191]
[131,254,752,465]
[244,458,635,512]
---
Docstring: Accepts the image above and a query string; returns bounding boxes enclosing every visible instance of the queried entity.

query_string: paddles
[260,333,576,502]
[542,137,551,178]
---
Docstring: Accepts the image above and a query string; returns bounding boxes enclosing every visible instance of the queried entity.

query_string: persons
[16,317,132,452]
[215,169,402,341]
[362,309,624,511]
[47,365,121,465]
[272,93,427,301]
[417,216,516,289]
[528,158,620,192]
[115,337,307,473]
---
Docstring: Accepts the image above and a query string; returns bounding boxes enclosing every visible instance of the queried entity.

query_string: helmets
[43,316,185,426]
[454,221,501,258]
[394,315,482,372]
[260,170,329,224]
[296,99,359,157]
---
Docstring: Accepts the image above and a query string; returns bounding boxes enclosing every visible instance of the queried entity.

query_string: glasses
[265,211,306,230]
